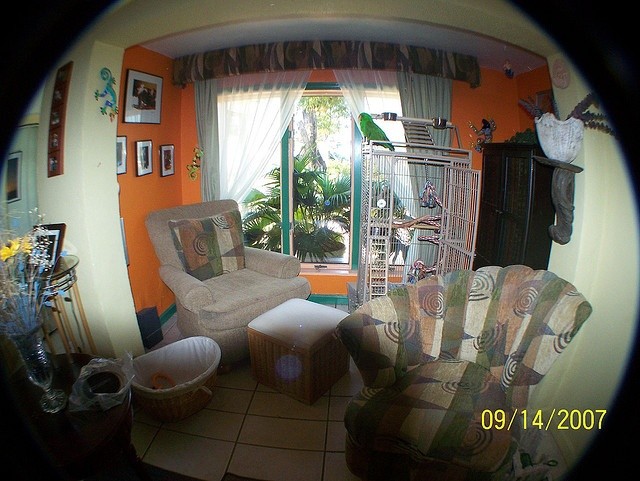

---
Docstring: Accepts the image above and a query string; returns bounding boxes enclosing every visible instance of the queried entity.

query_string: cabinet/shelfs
[471,141,556,270]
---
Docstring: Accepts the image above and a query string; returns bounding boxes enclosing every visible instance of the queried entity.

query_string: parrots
[357,112,396,152]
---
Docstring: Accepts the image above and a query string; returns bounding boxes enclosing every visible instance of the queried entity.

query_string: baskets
[120,335,221,423]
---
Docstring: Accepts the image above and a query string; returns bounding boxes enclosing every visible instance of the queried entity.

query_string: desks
[247,297,350,405]
[0,352,139,481]
[32,254,98,360]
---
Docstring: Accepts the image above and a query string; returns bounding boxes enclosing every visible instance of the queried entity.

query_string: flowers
[0,224,65,333]
[517,92,618,139]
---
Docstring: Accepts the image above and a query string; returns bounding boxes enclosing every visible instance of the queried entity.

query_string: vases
[8,325,69,413]
[533,113,585,164]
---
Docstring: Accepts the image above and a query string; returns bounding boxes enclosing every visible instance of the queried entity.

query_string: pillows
[168,209,246,281]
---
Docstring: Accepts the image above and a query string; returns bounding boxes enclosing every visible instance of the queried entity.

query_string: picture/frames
[47,61,73,177]
[5,149,22,203]
[160,144,175,177]
[122,67,163,125]
[136,138,154,177]
[117,134,128,176]
[32,224,66,274]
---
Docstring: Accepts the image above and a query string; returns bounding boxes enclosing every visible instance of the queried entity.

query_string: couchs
[144,200,312,370]
[334,264,593,481]
